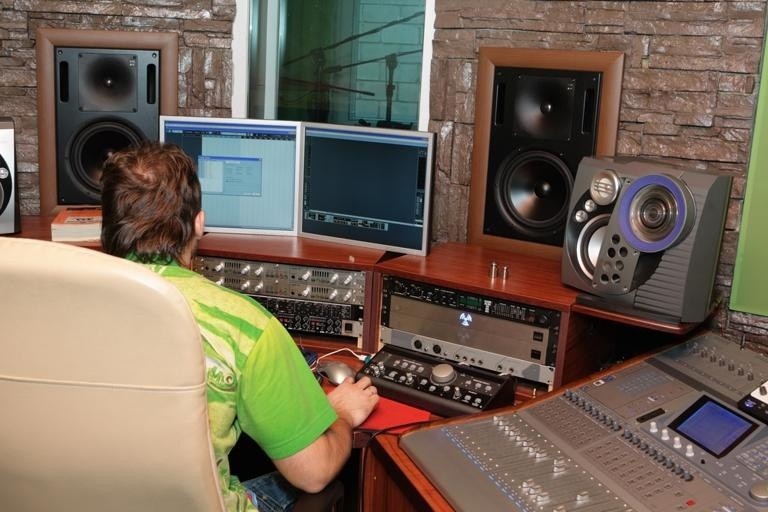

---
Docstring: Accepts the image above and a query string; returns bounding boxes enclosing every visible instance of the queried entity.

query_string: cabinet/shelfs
[0,231,726,512]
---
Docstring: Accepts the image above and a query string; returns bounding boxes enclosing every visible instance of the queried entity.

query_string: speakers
[561,153,733,325]
[0,117,18,234]
[54,46,160,205]
[482,65,601,248]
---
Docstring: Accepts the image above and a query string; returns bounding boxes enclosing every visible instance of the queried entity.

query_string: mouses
[317,361,355,385]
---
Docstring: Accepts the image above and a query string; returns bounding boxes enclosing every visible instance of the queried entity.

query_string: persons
[100,139,382,512]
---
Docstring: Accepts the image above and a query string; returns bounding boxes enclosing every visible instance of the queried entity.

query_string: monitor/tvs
[298,122,436,257]
[159,115,301,237]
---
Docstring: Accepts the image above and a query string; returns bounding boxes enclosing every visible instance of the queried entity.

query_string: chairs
[0,238,232,512]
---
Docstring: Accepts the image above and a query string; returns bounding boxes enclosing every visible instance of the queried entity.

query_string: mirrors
[232,0,438,133]
